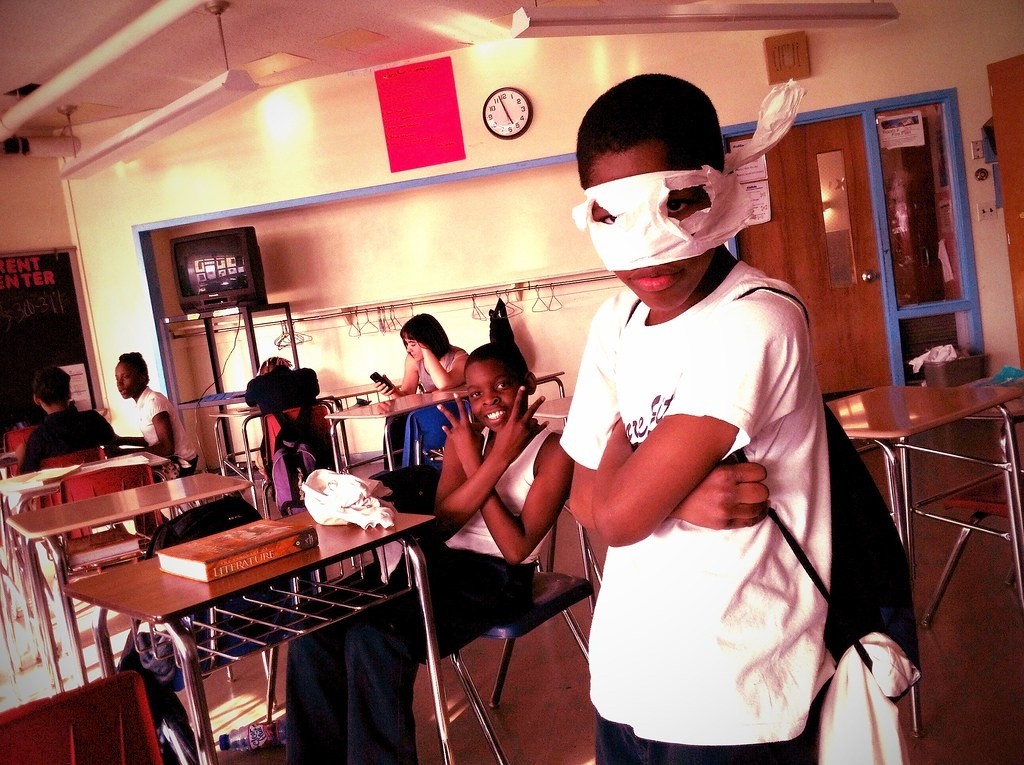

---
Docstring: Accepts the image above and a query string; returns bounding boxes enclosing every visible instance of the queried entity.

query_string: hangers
[273,282,563,350]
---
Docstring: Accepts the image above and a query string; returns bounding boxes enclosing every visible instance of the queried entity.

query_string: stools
[921,474,1023,629]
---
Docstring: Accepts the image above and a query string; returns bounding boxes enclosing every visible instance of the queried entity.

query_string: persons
[558,74,837,765]
[19,365,121,473]
[285,343,575,765]
[374,313,470,470]
[103,352,199,559]
[245,356,320,501]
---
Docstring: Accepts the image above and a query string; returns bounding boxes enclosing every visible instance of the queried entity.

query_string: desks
[0,380,1024,765]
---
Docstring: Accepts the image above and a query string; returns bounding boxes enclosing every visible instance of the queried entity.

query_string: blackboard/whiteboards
[0,246,107,457]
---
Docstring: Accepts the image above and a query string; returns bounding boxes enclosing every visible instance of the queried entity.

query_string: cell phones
[369,372,393,392]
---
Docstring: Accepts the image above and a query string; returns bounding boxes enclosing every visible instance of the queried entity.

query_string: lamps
[60,0,259,180]
[509,0,898,37]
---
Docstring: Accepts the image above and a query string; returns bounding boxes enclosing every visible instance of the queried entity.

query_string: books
[156,520,319,582]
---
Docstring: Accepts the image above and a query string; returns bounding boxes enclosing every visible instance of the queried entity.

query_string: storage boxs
[922,353,985,388]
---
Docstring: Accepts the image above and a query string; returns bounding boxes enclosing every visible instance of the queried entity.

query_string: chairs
[403,400,474,467]
[39,447,105,470]
[3,425,37,478]
[158,586,277,711]
[0,669,162,765]
[60,464,163,540]
[471,501,603,708]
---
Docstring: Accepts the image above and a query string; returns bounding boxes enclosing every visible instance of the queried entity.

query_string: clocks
[483,87,533,141]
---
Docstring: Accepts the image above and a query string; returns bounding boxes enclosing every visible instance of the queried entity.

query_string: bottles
[219,719,288,752]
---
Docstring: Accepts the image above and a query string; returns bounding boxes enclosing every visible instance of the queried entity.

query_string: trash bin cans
[923,352,986,391]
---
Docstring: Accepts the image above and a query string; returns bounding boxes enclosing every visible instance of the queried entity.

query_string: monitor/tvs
[169,226,269,314]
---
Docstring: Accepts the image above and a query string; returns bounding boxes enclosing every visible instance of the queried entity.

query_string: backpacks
[271,406,331,519]
[747,285,922,707]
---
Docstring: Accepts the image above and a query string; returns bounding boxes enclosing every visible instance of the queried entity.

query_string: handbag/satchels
[143,496,263,560]
